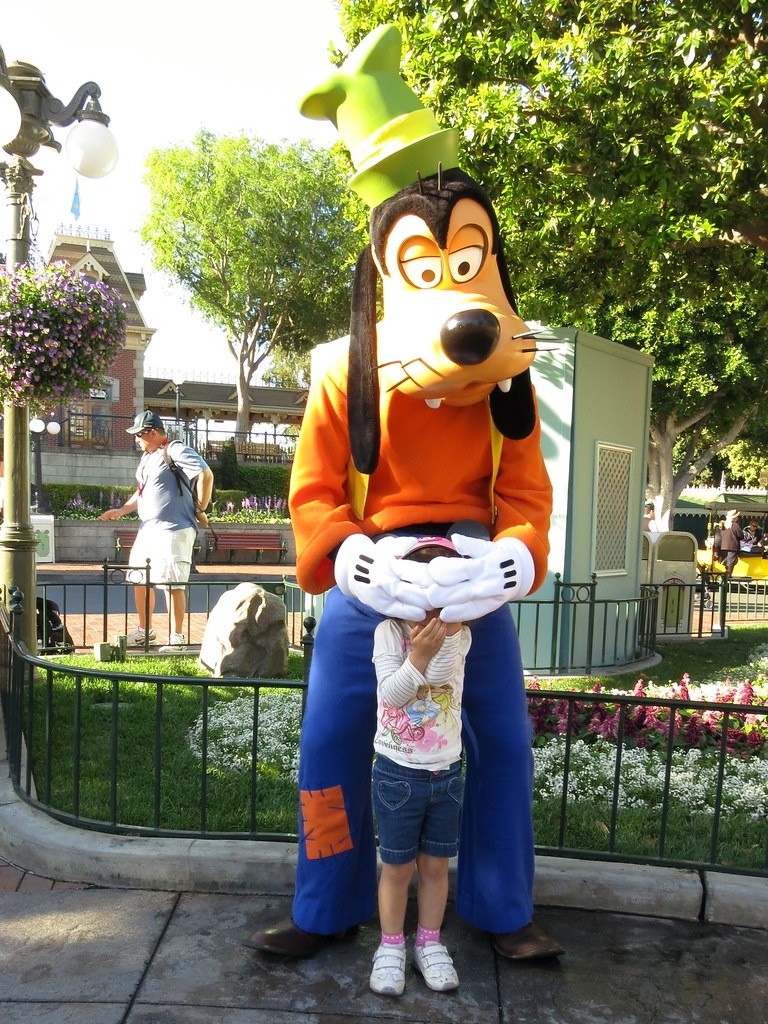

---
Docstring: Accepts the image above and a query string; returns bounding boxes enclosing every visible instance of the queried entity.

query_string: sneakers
[121,625,157,647]
[158,632,186,653]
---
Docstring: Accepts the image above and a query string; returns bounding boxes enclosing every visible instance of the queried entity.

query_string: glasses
[751,523,758,528]
[133,429,158,438]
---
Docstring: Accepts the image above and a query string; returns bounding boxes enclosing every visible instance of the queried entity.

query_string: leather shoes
[242,918,358,955]
[492,920,565,958]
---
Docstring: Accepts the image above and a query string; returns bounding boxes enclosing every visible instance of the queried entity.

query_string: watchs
[195,506,206,513]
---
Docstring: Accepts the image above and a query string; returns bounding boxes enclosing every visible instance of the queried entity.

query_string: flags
[71,177,81,222]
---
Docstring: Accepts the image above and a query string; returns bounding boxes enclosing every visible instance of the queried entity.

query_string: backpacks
[164,439,213,515]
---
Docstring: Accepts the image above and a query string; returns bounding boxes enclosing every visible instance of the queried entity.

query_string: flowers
[0,261,128,409]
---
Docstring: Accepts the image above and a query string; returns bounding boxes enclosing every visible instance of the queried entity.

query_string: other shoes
[413,940,460,992]
[369,941,406,997]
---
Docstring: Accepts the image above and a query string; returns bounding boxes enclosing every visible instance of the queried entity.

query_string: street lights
[1,43,121,690]
[28,414,60,515]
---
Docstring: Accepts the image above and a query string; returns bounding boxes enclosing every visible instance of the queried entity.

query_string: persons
[371,519,491,991]
[645,501,658,532]
[713,507,744,578]
[223,436,234,451]
[743,518,762,545]
[206,439,212,460]
[98,410,214,653]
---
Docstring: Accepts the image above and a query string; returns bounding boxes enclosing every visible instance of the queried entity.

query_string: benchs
[114,530,200,564]
[205,531,288,565]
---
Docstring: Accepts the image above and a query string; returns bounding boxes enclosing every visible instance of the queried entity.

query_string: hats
[723,509,740,529]
[398,535,465,559]
[125,409,164,435]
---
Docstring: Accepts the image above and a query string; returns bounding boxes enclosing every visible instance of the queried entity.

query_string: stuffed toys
[244,17,566,968]
